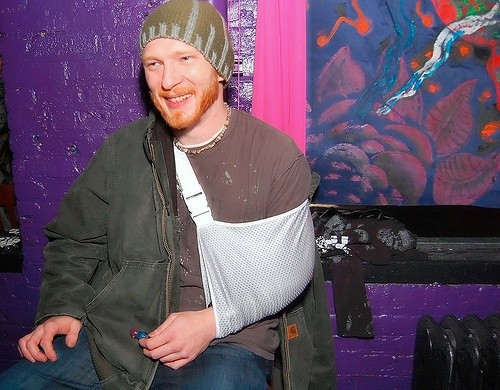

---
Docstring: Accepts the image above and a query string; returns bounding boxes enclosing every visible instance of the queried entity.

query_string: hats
[139,0,234,88]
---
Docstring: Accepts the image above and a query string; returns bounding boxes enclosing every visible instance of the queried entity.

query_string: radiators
[411,314,500,390]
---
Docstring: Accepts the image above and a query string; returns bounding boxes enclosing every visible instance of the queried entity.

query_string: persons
[0,0,336,390]
